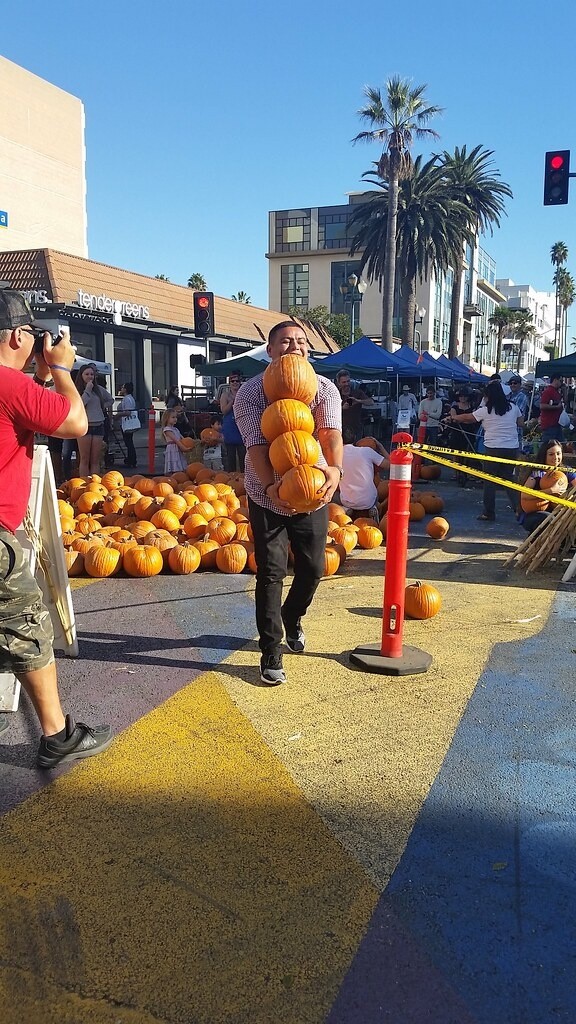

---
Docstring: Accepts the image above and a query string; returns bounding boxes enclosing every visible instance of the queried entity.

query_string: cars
[183,396,221,439]
[358,380,392,420]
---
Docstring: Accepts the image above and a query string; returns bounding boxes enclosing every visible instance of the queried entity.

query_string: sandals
[477,513,493,521]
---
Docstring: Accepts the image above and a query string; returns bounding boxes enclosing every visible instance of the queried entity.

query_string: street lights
[340,271,367,344]
[508,344,520,373]
[533,326,571,375]
[412,303,426,351]
[475,331,490,373]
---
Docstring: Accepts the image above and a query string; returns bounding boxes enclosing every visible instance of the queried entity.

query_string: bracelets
[264,482,274,496]
[50,365,71,373]
[33,372,55,387]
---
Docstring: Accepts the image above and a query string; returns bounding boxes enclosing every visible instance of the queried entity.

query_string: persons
[50,364,576,543]
[0,290,111,769]
[234,322,343,685]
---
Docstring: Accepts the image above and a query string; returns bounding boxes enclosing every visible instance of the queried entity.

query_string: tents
[27,352,113,447]
[195,333,576,426]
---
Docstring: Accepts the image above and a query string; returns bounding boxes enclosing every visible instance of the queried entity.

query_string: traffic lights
[543,150,570,206]
[193,292,216,338]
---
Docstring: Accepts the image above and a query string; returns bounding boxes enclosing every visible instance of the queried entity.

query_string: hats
[489,374,501,380]
[402,385,411,391]
[507,375,521,383]
[0,288,53,333]
[455,386,473,394]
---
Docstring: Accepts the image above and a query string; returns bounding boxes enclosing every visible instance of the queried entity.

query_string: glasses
[230,380,240,383]
[510,382,518,385]
[8,326,42,349]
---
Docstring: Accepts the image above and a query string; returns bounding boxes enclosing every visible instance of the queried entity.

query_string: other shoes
[124,459,137,468]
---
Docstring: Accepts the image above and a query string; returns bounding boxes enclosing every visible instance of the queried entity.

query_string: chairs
[183,409,199,439]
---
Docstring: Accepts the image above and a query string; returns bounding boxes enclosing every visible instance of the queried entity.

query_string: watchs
[330,463,344,482]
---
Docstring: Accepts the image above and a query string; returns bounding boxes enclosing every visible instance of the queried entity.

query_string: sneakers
[37,714,113,767]
[280,606,306,651]
[0,716,11,737]
[259,655,286,685]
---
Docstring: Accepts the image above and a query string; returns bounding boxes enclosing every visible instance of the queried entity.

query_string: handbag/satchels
[558,403,570,428]
[103,409,111,428]
[122,410,141,434]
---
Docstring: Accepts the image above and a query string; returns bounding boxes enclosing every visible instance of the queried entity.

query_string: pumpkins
[261,354,329,512]
[404,581,441,619]
[521,469,575,514]
[55,426,450,577]
